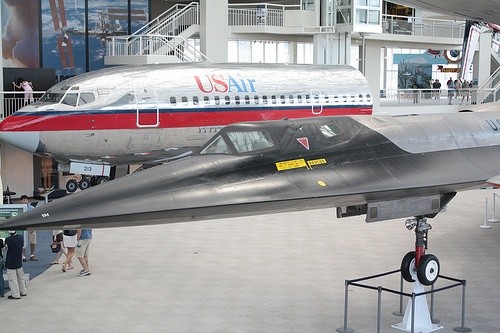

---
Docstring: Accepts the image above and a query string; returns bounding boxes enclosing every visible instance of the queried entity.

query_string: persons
[411,77,473,105]
[12,78,34,105]
[0,194,93,299]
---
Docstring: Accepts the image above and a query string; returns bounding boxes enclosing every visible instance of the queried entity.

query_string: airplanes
[0,100,500,286]
[0,61,374,193]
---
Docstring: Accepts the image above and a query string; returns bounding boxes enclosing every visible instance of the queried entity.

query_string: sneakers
[23,255,26,262]
[30,255,38,261]
[68,266,74,270]
[76,268,90,277]
[86,273,91,275]
[61,264,66,272]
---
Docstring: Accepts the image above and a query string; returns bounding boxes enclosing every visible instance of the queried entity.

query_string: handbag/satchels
[49,243,61,253]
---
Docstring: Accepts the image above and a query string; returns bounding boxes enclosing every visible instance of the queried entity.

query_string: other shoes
[63,261,72,264]
[50,261,59,264]
[8,295,20,299]
[20,293,27,296]
